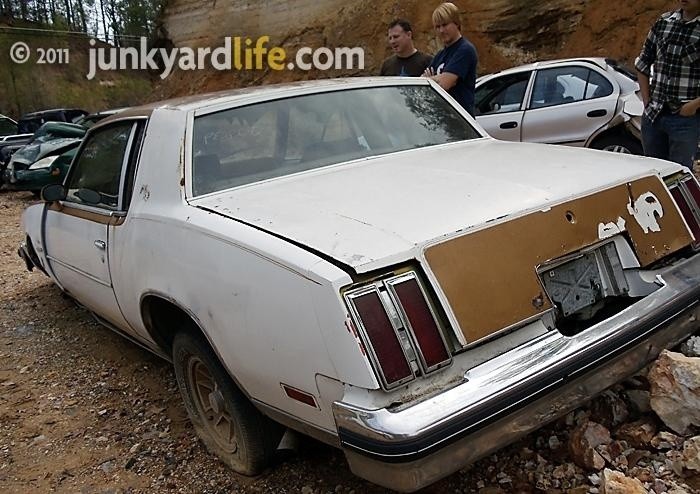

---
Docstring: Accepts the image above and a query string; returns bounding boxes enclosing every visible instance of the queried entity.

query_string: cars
[0,57,700,494]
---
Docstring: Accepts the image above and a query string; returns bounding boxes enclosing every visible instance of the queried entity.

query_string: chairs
[298,136,361,165]
[193,154,222,184]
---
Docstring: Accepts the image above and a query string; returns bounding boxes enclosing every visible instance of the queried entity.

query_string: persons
[631,0,700,173]
[418,1,478,123]
[378,15,434,78]
